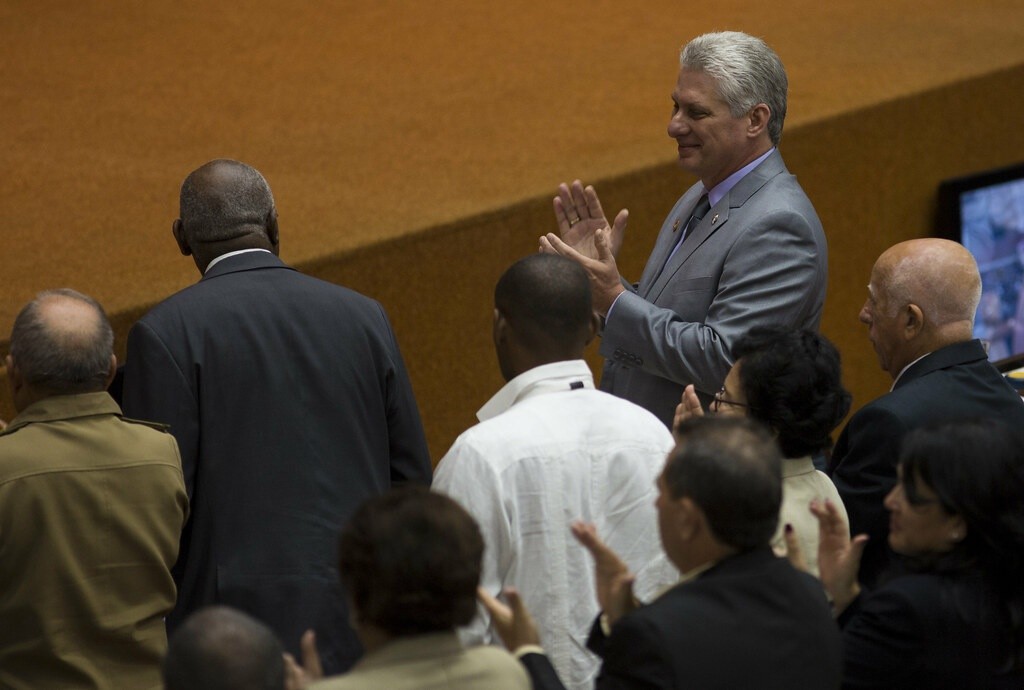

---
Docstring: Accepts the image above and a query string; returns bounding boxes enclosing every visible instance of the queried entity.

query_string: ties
[681,193,711,245]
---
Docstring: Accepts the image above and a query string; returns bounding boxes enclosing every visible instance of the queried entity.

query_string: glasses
[713,385,754,412]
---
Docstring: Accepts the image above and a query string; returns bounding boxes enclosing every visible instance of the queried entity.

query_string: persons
[539,32,830,437]
[0,165,1024,690]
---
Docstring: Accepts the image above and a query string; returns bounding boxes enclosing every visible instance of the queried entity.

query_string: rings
[569,215,581,226]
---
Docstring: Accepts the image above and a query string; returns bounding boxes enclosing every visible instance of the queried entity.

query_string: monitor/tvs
[932,162,1024,397]
